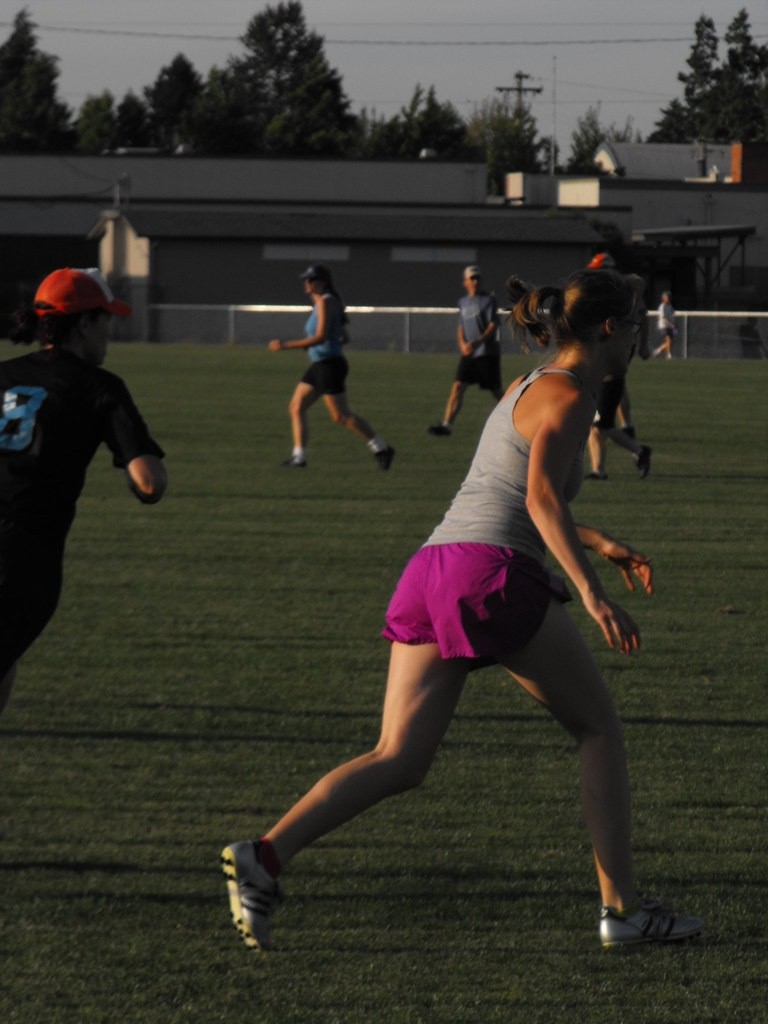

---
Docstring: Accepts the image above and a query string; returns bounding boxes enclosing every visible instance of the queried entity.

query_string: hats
[297,265,331,283]
[587,253,617,270]
[33,269,133,323]
[463,266,481,282]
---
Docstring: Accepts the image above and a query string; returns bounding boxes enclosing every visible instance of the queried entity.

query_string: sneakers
[221,838,289,954]
[599,897,706,949]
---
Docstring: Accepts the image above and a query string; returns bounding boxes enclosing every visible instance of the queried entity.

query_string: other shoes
[280,457,308,467]
[426,424,453,435]
[634,445,652,478]
[623,425,636,438]
[582,472,608,482]
[376,445,394,470]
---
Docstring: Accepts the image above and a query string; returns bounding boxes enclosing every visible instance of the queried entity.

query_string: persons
[581,254,653,482]
[428,265,504,437]
[220,270,716,960]
[267,265,396,472]
[0,268,167,716]
[653,289,676,360]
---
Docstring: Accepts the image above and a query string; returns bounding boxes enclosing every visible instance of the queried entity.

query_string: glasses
[304,277,319,286]
